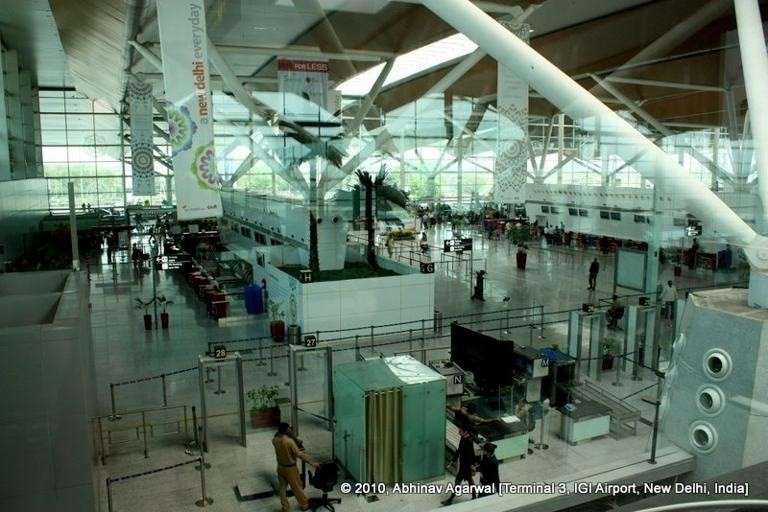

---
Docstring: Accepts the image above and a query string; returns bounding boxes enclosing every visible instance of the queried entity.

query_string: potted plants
[267,300,285,343]
[134,296,154,330]
[506,225,529,269]
[157,295,174,328]
[603,339,621,369]
[246,386,281,429]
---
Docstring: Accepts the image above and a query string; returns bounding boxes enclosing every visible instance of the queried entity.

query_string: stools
[299,270,313,283]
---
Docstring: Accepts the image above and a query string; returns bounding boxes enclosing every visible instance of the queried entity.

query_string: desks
[555,400,612,446]
[446,406,528,461]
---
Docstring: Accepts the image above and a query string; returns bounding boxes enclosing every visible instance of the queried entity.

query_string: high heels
[440,498,453,505]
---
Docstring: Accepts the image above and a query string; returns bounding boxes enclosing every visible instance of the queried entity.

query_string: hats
[483,443,497,450]
[460,425,469,432]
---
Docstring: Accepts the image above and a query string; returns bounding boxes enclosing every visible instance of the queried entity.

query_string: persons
[604,295,622,324]
[151,240,158,261]
[658,280,679,321]
[385,234,396,258]
[274,422,321,512]
[180,218,218,233]
[134,212,170,242]
[513,396,535,418]
[460,402,501,423]
[440,424,478,506]
[587,257,600,292]
[472,442,503,498]
[470,269,487,303]
[687,237,699,269]
[417,202,566,245]
[420,231,430,256]
[131,243,139,269]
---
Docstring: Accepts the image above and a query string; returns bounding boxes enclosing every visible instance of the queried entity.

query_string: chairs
[607,307,623,331]
[308,463,341,512]
[454,410,469,427]
[527,408,535,444]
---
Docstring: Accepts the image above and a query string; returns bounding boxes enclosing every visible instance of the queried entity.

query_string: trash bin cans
[288,325,301,345]
[245,284,263,314]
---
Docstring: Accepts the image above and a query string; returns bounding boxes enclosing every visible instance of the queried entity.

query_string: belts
[278,463,296,467]
